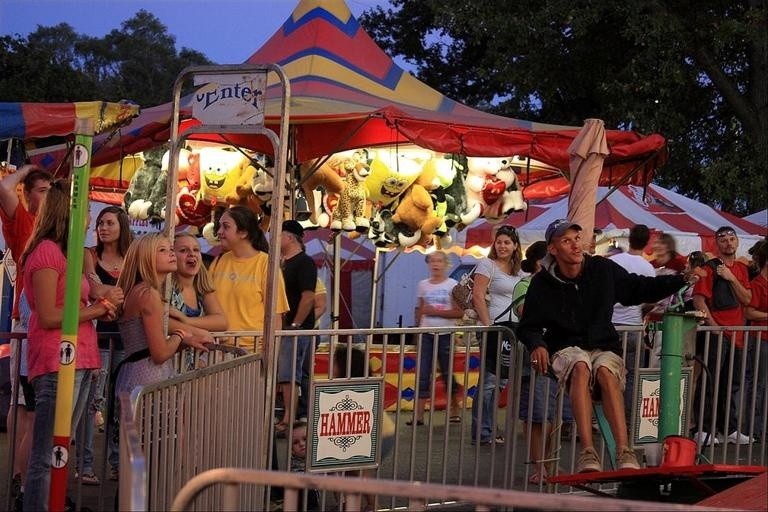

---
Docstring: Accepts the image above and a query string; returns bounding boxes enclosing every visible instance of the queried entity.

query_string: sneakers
[717,430,755,445]
[694,431,719,446]
[75,467,100,485]
[577,447,600,473]
[616,447,640,469]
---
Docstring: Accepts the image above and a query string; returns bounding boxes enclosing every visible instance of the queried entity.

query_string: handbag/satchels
[476,321,534,378]
[451,273,490,318]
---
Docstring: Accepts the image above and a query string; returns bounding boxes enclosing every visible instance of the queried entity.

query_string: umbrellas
[563,115,612,252]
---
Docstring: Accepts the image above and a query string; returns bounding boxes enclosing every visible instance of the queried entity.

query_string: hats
[268,220,303,236]
[545,219,582,244]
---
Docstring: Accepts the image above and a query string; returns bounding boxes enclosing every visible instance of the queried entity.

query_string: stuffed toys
[123,140,529,247]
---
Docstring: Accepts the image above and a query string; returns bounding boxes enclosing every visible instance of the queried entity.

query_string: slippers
[529,467,548,484]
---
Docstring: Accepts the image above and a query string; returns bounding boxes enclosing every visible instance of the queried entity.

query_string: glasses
[717,230,735,237]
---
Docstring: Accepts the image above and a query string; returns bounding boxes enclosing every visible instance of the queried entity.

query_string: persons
[403,250,470,423]
[473,220,767,486]
[1,160,393,512]
